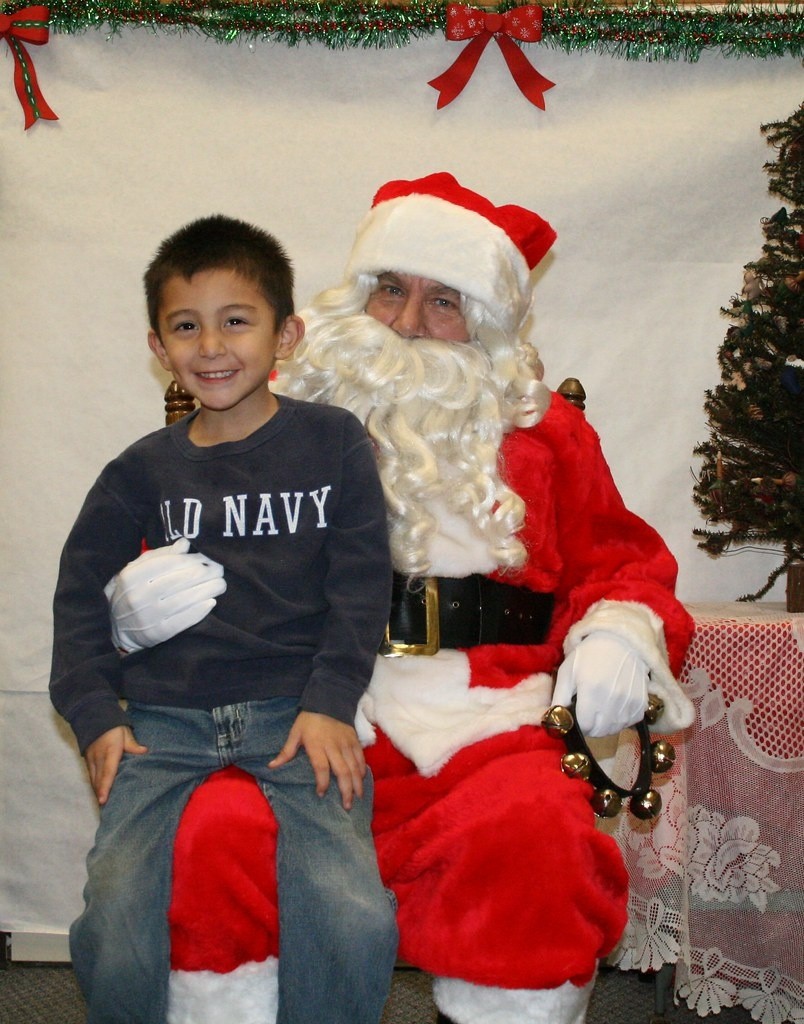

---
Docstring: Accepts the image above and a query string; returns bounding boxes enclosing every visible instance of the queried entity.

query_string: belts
[378,565,553,658]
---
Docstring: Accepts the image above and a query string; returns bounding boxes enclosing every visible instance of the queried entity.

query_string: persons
[47,215,399,1024]
[103,172,695,1024]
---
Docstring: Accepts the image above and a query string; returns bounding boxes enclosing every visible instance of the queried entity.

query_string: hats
[343,171,556,345]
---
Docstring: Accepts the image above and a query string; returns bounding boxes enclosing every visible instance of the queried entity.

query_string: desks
[593,602,804,1024]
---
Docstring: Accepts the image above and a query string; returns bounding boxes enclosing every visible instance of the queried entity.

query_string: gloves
[549,633,652,738]
[102,537,228,659]
